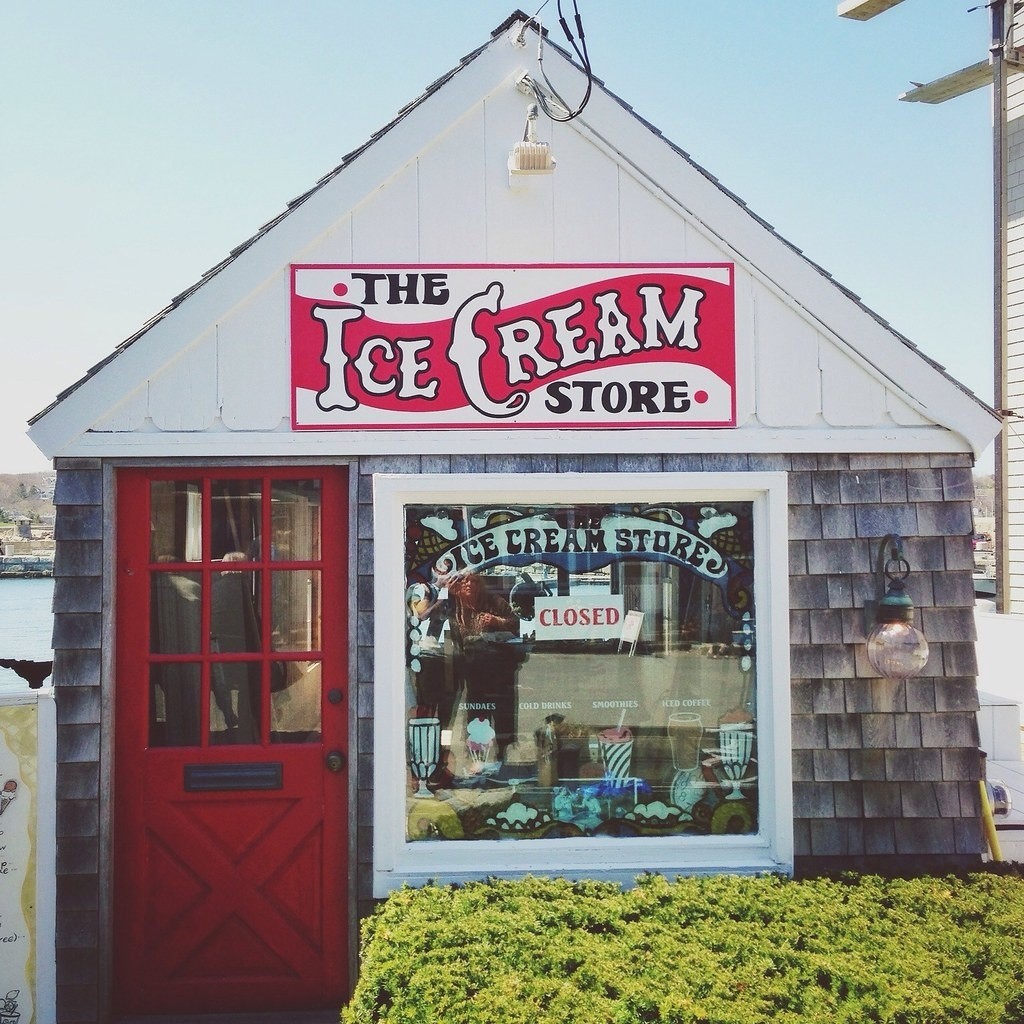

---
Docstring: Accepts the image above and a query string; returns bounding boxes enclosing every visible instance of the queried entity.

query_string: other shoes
[410,777,419,792]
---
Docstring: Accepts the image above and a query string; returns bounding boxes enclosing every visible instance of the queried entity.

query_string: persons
[207,553,281,729]
[148,554,202,746]
[405,554,519,778]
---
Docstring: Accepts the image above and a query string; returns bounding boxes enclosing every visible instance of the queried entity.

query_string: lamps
[864,534,929,682]
[507,103,557,191]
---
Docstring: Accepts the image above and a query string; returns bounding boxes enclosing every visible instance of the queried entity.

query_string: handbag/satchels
[254,660,303,692]
[462,613,526,664]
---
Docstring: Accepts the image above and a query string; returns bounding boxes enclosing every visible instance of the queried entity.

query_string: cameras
[450,574,464,583]
